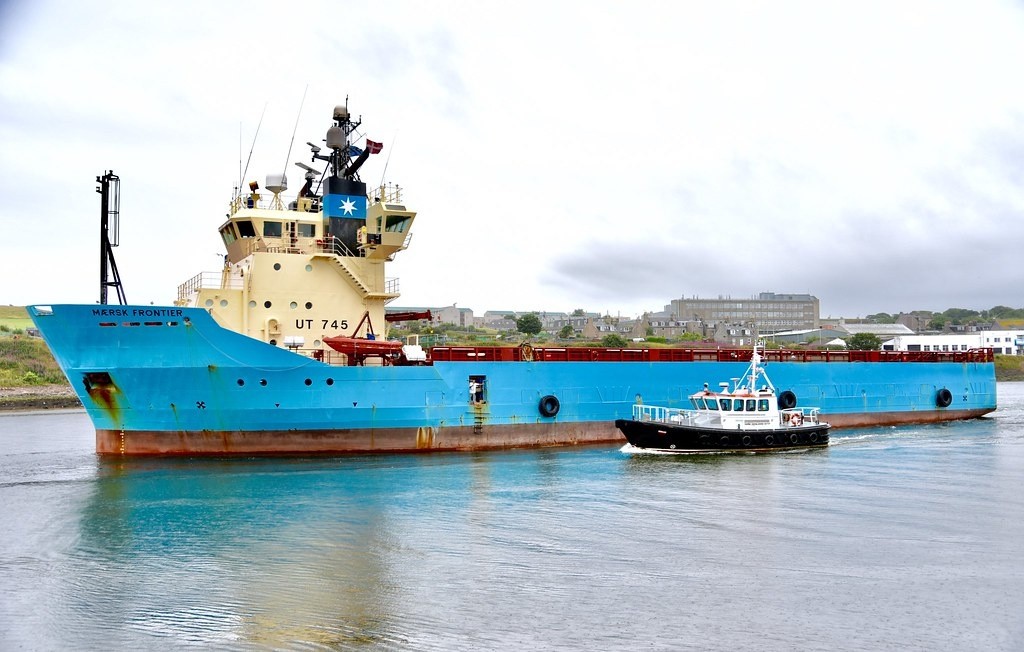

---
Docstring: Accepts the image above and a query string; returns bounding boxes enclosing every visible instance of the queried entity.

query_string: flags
[349,146,363,157]
[366,139,383,154]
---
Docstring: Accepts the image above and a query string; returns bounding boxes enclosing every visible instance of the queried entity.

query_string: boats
[321,335,403,356]
[26,79,997,455]
[615,338,831,452]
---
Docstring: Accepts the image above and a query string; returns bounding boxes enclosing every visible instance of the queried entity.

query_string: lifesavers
[779,391,797,409]
[540,395,560,417]
[809,432,818,443]
[765,435,774,446]
[790,434,798,444]
[791,414,801,425]
[742,436,751,447]
[720,436,730,446]
[699,435,710,445]
[936,389,953,406]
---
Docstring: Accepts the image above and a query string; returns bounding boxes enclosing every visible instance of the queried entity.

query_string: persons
[470,379,484,403]
